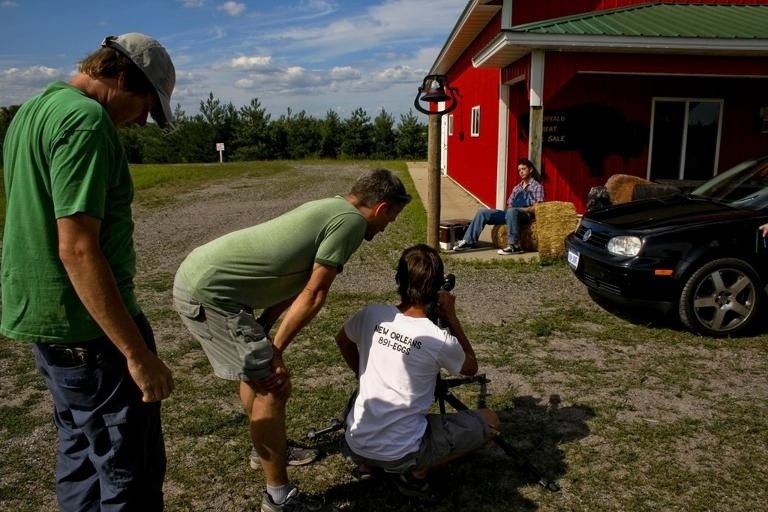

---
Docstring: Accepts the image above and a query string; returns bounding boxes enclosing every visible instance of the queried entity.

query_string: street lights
[414,73,457,253]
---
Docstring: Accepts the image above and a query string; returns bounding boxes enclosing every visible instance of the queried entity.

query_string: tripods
[307,374,560,492]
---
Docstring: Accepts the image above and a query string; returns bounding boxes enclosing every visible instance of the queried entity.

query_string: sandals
[350,465,377,482]
[394,470,443,502]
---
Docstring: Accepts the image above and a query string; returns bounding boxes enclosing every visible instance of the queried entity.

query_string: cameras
[441,273,457,291]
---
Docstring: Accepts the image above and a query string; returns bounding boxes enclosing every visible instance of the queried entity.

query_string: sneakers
[261,485,338,512]
[497,244,523,255]
[250,439,320,470]
[453,240,471,251]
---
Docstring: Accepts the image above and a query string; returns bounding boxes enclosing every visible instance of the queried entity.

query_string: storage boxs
[439,219,473,250]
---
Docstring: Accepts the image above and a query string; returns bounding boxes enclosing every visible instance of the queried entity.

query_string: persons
[332,242,501,497]
[0,33,177,511]
[171,168,413,511]
[452,159,545,255]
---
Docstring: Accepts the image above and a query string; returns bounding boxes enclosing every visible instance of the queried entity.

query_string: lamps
[414,74,464,116]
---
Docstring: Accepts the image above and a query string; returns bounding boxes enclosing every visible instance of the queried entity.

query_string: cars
[564,155,768,338]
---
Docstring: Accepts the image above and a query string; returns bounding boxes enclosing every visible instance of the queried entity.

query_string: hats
[99,32,175,129]
